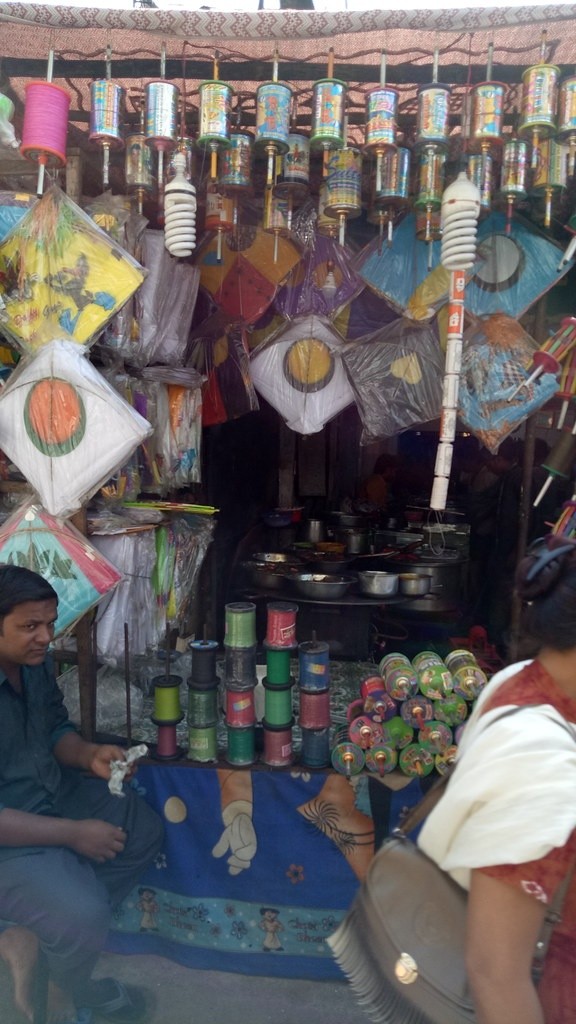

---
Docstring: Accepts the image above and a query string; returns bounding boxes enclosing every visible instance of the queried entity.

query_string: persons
[361,454,397,505]
[401,436,576,635]
[415,558,576,1024]
[179,460,310,604]
[1,563,164,1023]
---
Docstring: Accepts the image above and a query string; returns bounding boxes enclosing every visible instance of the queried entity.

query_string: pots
[307,517,341,546]
[329,527,377,554]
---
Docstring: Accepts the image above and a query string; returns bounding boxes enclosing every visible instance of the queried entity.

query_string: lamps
[440,30,482,269]
[164,42,198,258]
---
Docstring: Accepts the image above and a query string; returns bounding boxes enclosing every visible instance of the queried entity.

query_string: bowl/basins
[359,571,399,599]
[285,574,358,600]
[301,552,356,575]
[261,512,293,527]
[340,516,373,527]
[253,553,309,567]
[383,555,470,593]
[399,573,433,596]
[317,542,347,560]
[275,507,305,522]
[240,562,300,589]
